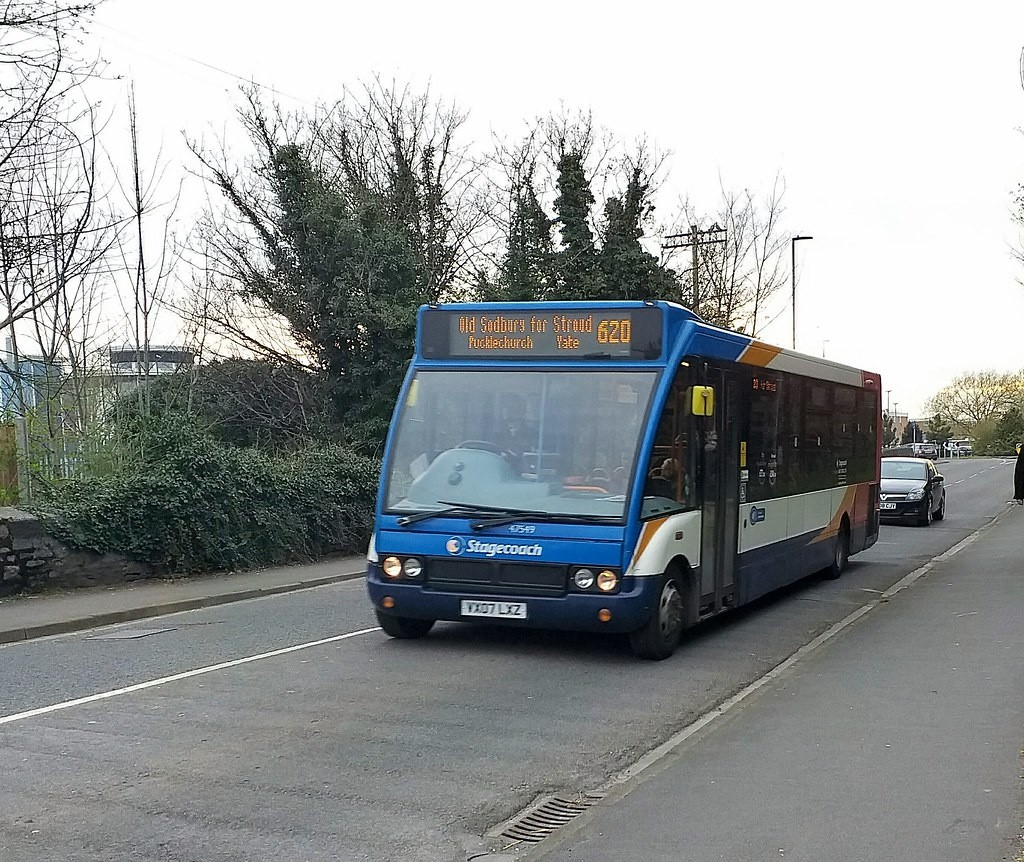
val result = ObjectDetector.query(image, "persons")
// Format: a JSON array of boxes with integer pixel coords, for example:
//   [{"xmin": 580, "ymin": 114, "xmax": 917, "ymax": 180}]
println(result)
[
  {"xmin": 595, "ymin": 447, "xmax": 689, "ymax": 501},
  {"xmin": 456, "ymin": 395, "xmax": 547, "ymax": 474}
]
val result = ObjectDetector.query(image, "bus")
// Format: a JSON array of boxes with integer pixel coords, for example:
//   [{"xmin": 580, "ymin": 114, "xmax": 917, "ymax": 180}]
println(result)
[{"xmin": 361, "ymin": 297, "xmax": 884, "ymax": 664}]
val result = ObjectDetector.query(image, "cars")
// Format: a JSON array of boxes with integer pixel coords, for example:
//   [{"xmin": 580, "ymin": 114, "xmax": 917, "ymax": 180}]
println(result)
[
  {"xmin": 915, "ymin": 447, "xmax": 937, "ymax": 461},
  {"xmin": 879, "ymin": 457, "xmax": 947, "ymax": 527}
]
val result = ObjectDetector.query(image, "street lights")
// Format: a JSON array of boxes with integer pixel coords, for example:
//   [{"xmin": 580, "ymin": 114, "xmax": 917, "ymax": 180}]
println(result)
[
  {"xmin": 885, "ymin": 390, "xmax": 891, "ymax": 415},
  {"xmin": 790, "ymin": 235, "xmax": 813, "ymax": 349},
  {"xmin": 893, "ymin": 403, "xmax": 898, "ymax": 423},
  {"xmin": 822, "ymin": 339, "xmax": 829, "ymax": 358}
]
